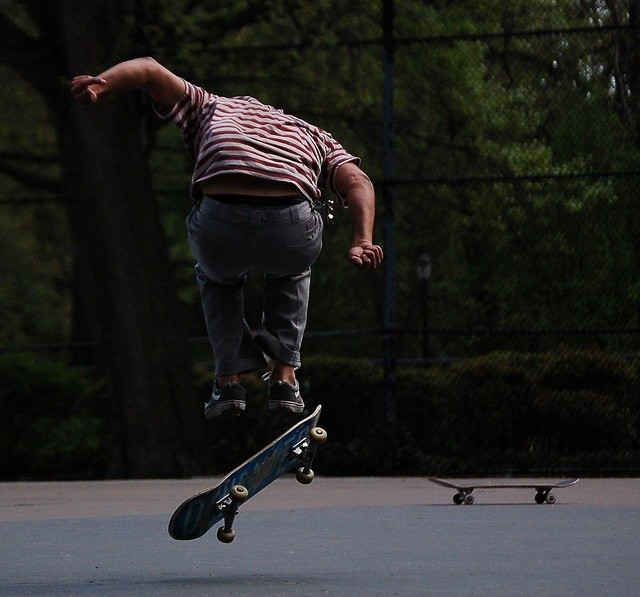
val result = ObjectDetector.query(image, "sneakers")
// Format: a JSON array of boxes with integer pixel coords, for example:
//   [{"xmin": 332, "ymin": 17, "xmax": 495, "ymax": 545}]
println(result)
[
  {"xmin": 260, "ymin": 371, "xmax": 305, "ymax": 412},
  {"xmin": 204, "ymin": 379, "xmax": 247, "ymax": 419}
]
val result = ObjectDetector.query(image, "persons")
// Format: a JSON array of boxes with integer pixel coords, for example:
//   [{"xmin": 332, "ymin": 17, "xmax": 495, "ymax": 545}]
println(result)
[{"xmin": 71, "ymin": 55, "xmax": 383, "ymax": 419}]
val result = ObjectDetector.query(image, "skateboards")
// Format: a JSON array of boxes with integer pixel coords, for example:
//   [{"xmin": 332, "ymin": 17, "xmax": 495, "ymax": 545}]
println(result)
[
  {"xmin": 428, "ymin": 476, "xmax": 579, "ymax": 504},
  {"xmin": 168, "ymin": 405, "xmax": 327, "ymax": 543}
]
